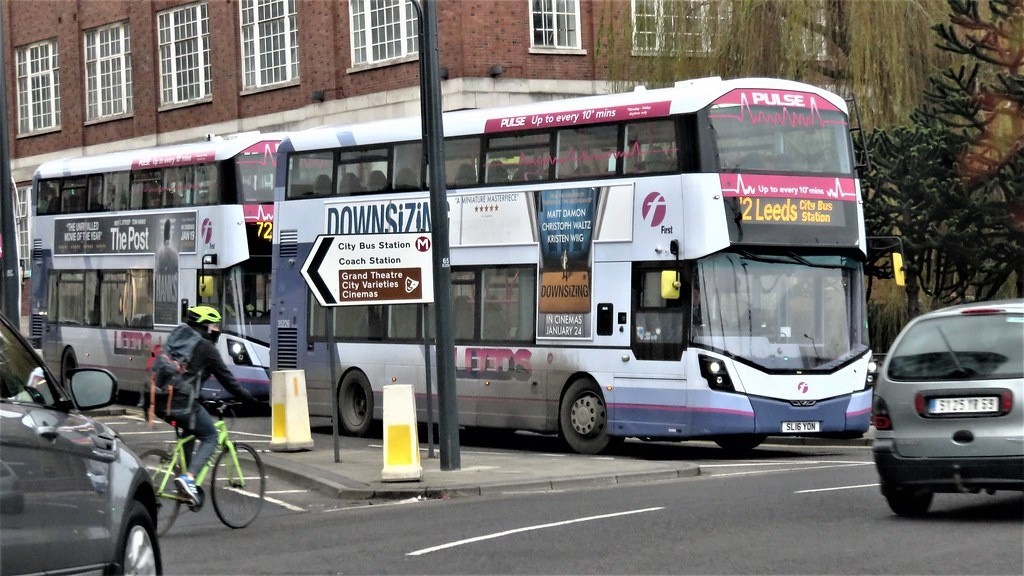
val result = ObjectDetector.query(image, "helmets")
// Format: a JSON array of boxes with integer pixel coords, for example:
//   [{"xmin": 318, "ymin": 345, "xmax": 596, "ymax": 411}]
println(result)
[{"xmin": 188, "ymin": 305, "xmax": 222, "ymax": 323}]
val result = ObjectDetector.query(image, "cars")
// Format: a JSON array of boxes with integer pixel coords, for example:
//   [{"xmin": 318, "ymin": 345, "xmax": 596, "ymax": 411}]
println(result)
[
  {"xmin": 870, "ymin": 299, "xmax": 1024, "ymax": 518},
  {"xmin": 0, "ymin": 311, "xmax": 162, "ymax": 576}
]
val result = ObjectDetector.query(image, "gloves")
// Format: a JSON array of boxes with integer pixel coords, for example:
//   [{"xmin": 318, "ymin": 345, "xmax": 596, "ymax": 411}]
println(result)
[{"xmin": 235, "ymin": 388, "xmax": 252, "ymax": 406}]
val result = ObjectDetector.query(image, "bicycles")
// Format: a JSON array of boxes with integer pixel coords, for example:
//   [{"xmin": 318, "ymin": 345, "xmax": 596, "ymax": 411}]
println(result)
[{"xmin": 138, "ymin": 399, "xmax": 265, "ymax": 539}]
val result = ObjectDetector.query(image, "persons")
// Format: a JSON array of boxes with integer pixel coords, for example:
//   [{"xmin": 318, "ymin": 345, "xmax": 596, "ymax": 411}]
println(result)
[
  {"xmin": 153, "ymin": 305, "xmax": 253, "ymax": 504},
  {"xmin": 157, "ymin": 218, "xmax": 178, "ymax": 275},
  {"xmin": 693, "ymin": 286, "xmax": 702, "ymax": 327},
  {"xmin": 561, "ymin": 249, "xmax": 569, "ymax": 281}
]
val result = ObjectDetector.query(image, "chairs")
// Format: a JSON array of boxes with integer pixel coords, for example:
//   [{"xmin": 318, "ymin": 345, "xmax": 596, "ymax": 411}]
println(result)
[
  {"xmin": 396, "ymin": 169, "xmax": 420, "ymax": 188},
  {"xmin": 314, "ymin": 174, "xmax": 332, "ymax": 194},
  {"xmin": 454, "ymin": 163, "xmax": 476, "ymax": 185},
  {"xmin": 487, "ymin": 160, "xmax": 509, "ymax": 183},
  {"xmin": 646, "ymin": 147, "xmax": 672, "ymax": 172},
  {"xmin": 340, "ymin": 173, "xmax": 361, "ymax": 194},
  {"xmin": 367, "ymin": 171, "xmax": 386, "ymax": 191}
]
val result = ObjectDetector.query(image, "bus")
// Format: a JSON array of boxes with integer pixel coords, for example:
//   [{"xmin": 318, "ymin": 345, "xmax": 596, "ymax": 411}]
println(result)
[
  {"xmin": 27, "ymin": 130, "xmax": 290, "ymax": 402},
  {"xmin": 267, "ymin": 74, "xmax": 873, "ymax": 458}
]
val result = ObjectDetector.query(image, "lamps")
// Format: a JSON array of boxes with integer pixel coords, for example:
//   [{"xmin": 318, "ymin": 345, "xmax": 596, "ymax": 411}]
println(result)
[
  {"xmin": 440, "ymin": 68, "xmax": 449, "ymax": 80},
  {"xmin": 309, "ymin": 90, "xmax": 325, "ymax": 102},
  {"xmin": 489, "ymin": 65, "xmax": 503, "ymax": 79}
]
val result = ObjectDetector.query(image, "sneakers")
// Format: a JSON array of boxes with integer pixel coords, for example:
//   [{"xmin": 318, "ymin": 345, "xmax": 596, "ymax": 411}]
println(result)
[{"xmin": 174, "ymin": 474, "xmax": 201, "ymax": 505}]
